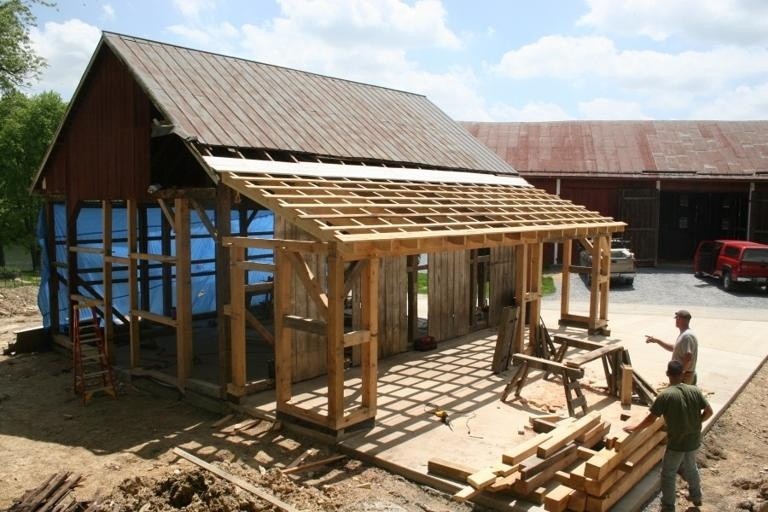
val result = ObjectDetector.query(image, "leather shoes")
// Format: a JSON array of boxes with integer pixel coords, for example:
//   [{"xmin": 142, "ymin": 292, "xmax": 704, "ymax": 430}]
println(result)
[{"xmin": 684, "ymin": 492, "xmax": 701, "ymax": 506}]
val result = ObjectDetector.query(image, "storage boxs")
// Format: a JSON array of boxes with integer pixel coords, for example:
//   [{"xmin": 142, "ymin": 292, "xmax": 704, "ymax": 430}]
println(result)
[{"xmin": 164, "ymin": 341, "xmax": 201, "ymax": 378}]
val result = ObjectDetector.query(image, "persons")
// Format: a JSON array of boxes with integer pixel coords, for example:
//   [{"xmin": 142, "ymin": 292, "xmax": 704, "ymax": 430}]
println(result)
[
  {"xmin": 623, "ymin": 360, "xmax": 713, "ymax": 505},
  {"xmin": 645, "ymin": 310, "xmax": 698, "ymax": 385}
]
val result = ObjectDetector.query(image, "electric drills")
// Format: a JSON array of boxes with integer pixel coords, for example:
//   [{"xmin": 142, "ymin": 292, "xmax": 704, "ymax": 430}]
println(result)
[{"xmin": 435, "ymin": 410, "xmax": 455, "ymax": 432}]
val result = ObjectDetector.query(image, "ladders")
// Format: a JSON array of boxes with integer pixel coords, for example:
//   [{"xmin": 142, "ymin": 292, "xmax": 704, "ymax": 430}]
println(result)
[{"xmin": 72, "ymin": 301, "xmax": 119, "ymax": 406}]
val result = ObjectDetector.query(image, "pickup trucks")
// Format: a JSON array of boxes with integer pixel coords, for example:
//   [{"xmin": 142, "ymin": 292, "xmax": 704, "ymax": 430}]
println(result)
[{"xmin": 577, "ymin": 239, "xmax": 638, "ymax": 289}]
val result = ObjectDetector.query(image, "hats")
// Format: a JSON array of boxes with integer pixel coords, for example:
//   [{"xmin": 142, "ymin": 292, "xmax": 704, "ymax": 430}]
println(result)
[{"xmin": 675, "ymin": 310, "xmax": 691, "ymax": 319}]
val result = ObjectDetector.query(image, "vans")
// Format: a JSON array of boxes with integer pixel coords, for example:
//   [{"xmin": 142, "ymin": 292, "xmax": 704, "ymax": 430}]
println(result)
[{"xmin": 692, "ymin": 238, "xmax": 768, "ymax": 295}]
